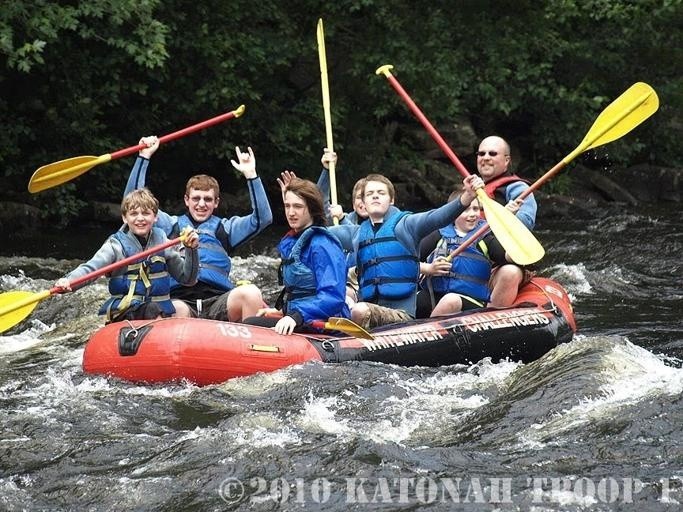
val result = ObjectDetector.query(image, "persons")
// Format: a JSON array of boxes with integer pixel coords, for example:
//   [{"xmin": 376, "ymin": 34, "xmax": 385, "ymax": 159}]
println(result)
[{"xmin": 53, "ymin": 136, "xmax": 538, "ymax": 336}]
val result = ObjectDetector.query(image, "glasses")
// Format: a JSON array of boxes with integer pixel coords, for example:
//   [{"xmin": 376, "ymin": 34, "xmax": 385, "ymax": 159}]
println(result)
[
  {"xmin": 187, "ymin": 195, "xmax": 215, "ymax": 203},
  {"xmin": 475, "ymin": 150, "xmax": 507, "ymax": 157}
]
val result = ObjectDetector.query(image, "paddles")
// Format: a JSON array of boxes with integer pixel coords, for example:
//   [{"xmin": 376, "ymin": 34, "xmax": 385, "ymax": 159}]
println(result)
[
  {"xmin": 0, "ymin": 235, "xmax": 188, "ymax": 333},
  {"xmin": 262, "ymin": 313, "xmax": 374, "ymax": 340},
  {"xmin": 375, "ymin": 64, "xmax": 545, "ymax": 266},
  {"xmin": 314, "ymin": 18, "xmax": 338, "ymax": 225},
  {"xmin": 28, "ymin": 104, "xmax": 245, "ymax": 193},
  {"xmin": 446, "ymin": 81, "xmax": 660, "ymax": 264}
]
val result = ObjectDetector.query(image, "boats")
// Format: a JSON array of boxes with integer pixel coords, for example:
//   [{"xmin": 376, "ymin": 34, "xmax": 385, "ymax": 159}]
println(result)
[{"xmin": 82, "ymin": 277, "xmax": 577, "ymax": 385}]
